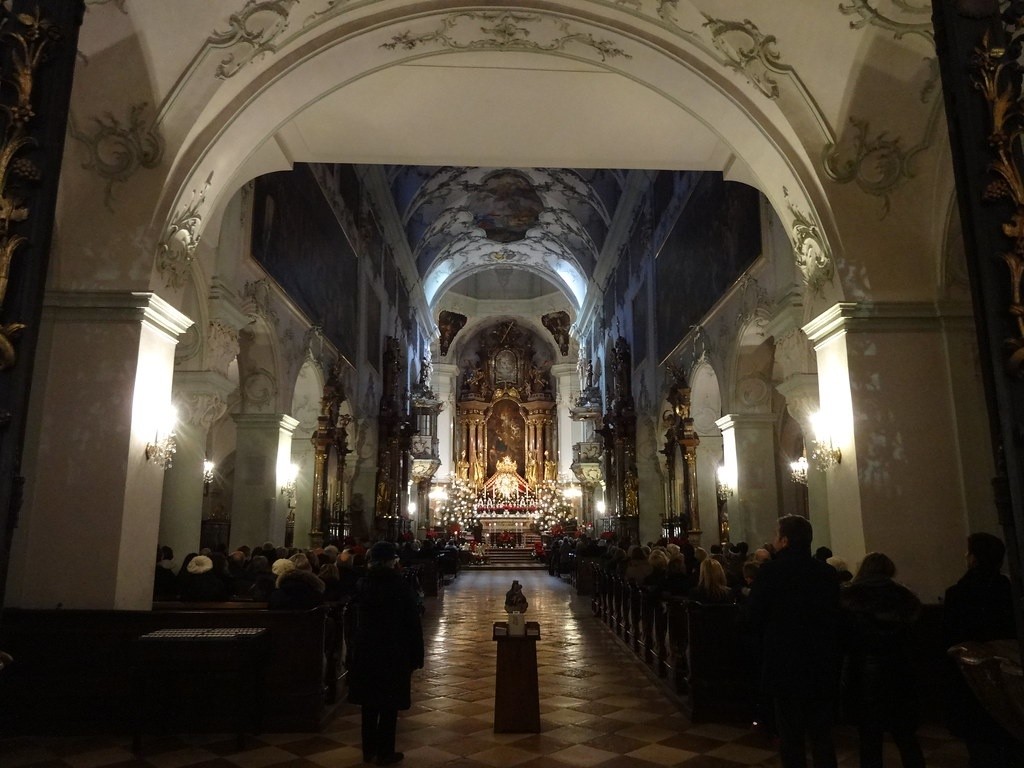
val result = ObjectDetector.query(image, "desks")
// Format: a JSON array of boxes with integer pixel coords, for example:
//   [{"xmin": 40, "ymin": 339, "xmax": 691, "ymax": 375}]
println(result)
[{"xmin": 490, "ymin": 532, "xmax": 522, "ymax": 548}]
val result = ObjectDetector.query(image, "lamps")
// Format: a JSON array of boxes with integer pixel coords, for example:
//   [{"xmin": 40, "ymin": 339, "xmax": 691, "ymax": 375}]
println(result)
[
  {"xmin": 281, "ymin": 480, "xmax": 295, "ymax": 494},
  {"xmin": 146, "ymin": 435, "xmax": 177, "ymax": 471},
  {"xmin": 812, "ymin": 437, "xmax": 841, "ymax": 471},
  {"xmin": 718, "ymin": 480, "xmax": 733, "ymax": 502}
]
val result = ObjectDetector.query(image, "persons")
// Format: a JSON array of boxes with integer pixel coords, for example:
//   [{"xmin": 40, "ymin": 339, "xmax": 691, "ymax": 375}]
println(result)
[
  {"xmin": 342, "ymin": 541, "xmax": 424, "ymax": 764},
  {"xmin": 747, "ymin": 512, "xmax": 848, "ymax": 768},
  {"xmin": 941, "ymin": 531, "xmax": 1024, "ymax": 768},
  {"xmin": 837, "ymin": 551, "xmax": 928, "ymax": 768},
  {"xmin": 155, "ymin": 525, "xmax": 777, "ymax": 704}
]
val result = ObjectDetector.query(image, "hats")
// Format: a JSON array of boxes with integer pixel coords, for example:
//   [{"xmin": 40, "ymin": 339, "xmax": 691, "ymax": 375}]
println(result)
[
  {"xmin": 272, "ymin": 558, "xmax": 295, "ymax": 575},
  {"xmin": 187, "ymin": 555, "xmax": 213, "ymax": 574}
]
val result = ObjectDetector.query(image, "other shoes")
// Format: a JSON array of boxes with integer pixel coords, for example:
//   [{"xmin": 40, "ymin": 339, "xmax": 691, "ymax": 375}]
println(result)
[{"xmin": 364, "ymin": 752, "xmax": 404, "ymax": 765}]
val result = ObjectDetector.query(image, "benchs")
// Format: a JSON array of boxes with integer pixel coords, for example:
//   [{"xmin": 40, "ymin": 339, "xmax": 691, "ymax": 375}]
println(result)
[
  {"xmin": 551, "ymin": 553, "xmax": 954, "ymax": 723},
  {"xmin": 0, "ymin": 552, "xmax": 461, "ymax": 733}
]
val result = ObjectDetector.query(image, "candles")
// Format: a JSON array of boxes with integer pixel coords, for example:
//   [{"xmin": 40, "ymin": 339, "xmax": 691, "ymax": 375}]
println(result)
[
  {"xmin": 484, "ymin": 485, "xmax": 486, "ymax": 499},
  {"xmin": 517, "ymin": 485, "xmax": 519, "ymax": 499},
  {"xmin": 526, "ymin": 485, "xmax": 528, "ymax": 500},
  {"xmin": 493, "ymin": 484, "xmax": 495, "ymax": 502},
  {"xmin": 475, "ymin": 483, "xmax": 477, "ymax": 497},
  {"xmin": 535, "ymin": 485, "xmax": 537, "ymax": 498}
]
